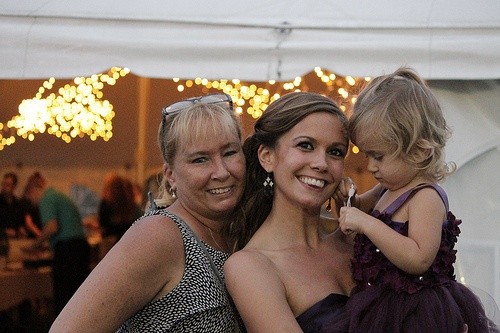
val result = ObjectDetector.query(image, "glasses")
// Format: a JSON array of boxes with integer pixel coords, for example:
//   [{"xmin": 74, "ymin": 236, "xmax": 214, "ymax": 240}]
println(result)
[{"xmin": 161, "ymin": 93, "xmax": 233, "ymax": 142}]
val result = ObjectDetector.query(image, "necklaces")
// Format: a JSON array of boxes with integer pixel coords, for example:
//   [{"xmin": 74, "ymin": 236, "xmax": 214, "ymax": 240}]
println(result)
[{"xmin": 208, "ymin": 227, "xmax": 226, "ymax": 253}]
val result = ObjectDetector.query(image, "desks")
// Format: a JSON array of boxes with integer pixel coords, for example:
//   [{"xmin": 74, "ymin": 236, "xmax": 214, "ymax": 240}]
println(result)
[{"xmin": 0, "ymin": 254, "xmax": 54, "ymax": 325}]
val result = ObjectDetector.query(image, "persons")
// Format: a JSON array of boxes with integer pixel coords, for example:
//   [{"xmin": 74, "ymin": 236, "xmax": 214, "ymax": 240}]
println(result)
[
  {"xmin": 224, "ymin": 93, "xmax": 358, "ymax": 333},
  {"xmin": 47, "ymin": 94, "xmax": 251, "ymax": 333},
  {"xmin": 329, "ymin": 66, "xmax": 490, "ymax": 333},
  {"xmin": 0, "ymin": 172, "xmax": 173, "ymax": 333}
]
